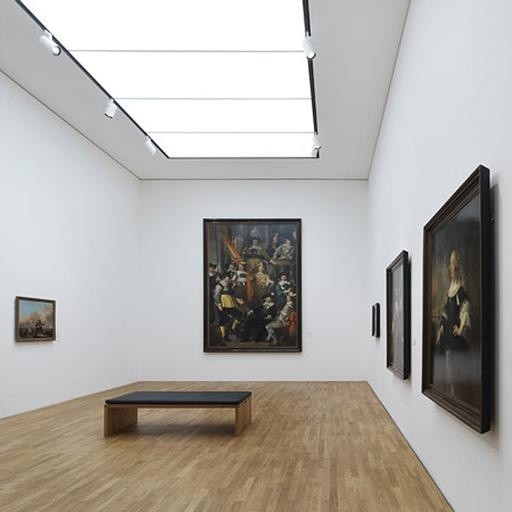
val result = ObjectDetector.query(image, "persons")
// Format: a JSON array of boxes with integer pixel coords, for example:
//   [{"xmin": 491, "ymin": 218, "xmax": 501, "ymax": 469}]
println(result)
[
  {"xmin": 429, "ymin": 246, "xmax": 471, "ymax": 401},
  {"xmin": 209, "ymin": 222, "xmax": 298, "ymax": 347}
]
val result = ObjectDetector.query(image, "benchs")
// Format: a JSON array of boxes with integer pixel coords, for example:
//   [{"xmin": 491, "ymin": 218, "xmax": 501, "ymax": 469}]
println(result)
[{"xmin": 103, "ymin": 391, "xmax": 252, "ymax": 436}]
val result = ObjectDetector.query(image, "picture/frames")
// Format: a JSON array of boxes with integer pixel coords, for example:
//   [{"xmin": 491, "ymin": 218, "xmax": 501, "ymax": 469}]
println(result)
[
  {"xmin": 201, "ymin": 218, "xmax": 303, "ymax": 354},
  {"xmin": 384, "ymin": 163, "xmax": 494, "ymax": 435},
  {"xmin": 14, "ymin": 295, "xmax": 57, "ymax": 343}
]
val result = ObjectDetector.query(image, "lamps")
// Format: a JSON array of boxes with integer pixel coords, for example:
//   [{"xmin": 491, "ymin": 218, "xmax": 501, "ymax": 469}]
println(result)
[
  {"xmin": 302, "ymin": 33, "xmax": 317, "ymax": 60},
  {"xmin": 311, "ymin": 130, "xmax": 323, "ymax": 152},
  {"xmin": 144, "ymin": 137, "xmax": 158, "ymax": 158},
  {"xmin": 105, "ymin": 97, "xmax": 118, "ymax": 120},
  {"xmin": 39, "ymin": 30, "xmax": 61, "ymax": 58}
]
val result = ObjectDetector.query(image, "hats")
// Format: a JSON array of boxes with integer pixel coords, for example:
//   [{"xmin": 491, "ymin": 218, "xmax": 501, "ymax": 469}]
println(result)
[
  {"xmin": 264, "ymin": 291, "xmax": 273, "ymax": 299},
  {"xmin": 209, "ymin": 260, "xmax": 246, "ymax": 279},
  {"xmin": 257, "ymin": 262, "xmax": 267, "ymax": 269},
  {"xmin": 279, "ymin": 272, "xmax": 289, "ymax": 278},
  {"xmin": 248, "ymin": 232, "xmax": 280, "ymax": 242}
]
